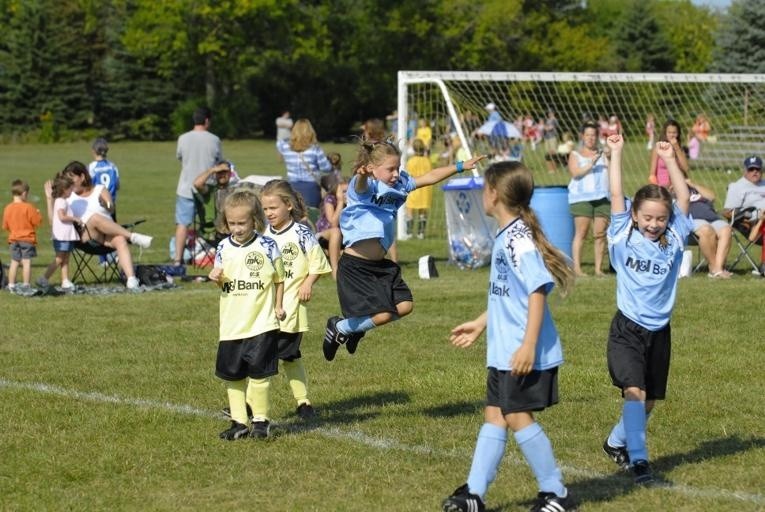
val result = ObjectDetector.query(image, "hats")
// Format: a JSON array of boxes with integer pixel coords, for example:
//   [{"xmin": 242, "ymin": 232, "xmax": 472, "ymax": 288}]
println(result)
[{"xmin": 744, "ymin": 156, "xmax": 762, "ymax": 169}]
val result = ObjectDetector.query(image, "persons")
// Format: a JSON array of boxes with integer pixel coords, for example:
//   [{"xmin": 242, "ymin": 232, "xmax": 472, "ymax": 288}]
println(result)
[
  {"xmin": 601, "ymin": 134, "xmax": 696, "ymax": 483},
  {"xmin": 691, "ymin": 114, "xmax": 711, "ymax": 142},
  {"xmin": 1, "ymin": 178, "xmax": 41, "ymax": 293},
  {"xmin": 193, "ymin": 159, "xmax": 234, "ymax": 248},
  {"xmin": 172, "ymin": 108, "xmax": 223, "ymax": 266},
  {"xmin": 323, "ymin": 141, "xmax": 489, "ymax": 362},
  {"xmin": 322, "ymin": 151, "xmax": 345, "ymax": 193},
  {"xmin": 648, "ymin": 119, "xmax": 689, "ymax": 189},
  {"xmin": 207, "ymin": 190, "xmax": 287, "ymax": 440},
  {"xmin": 35, "ymin": 175, "xmax": 82, "ymax": 290},
  {"xmin": 364, "ymin": 102, "xmax": 544, "ymax": 157},
  {"xmin": 544, "ymin": 109, "xmax": 558, "ymax": 151},
  {"xmin": 597, "ymin": 115, "xmax": 608, "ymax": 147},
  {"xmin": 404, "ymin": 138, "xmax": 433, "ymax": 240},
  {"xmin": 316, "ymin": 175, "xmax": 351, "ymax": 283},
  {"xmin": 220, "ymin": 179, "xmax": 333, "ymax": 419},
  {"xmin": 721, "ymin": 155, "xmax": 765, "ymax": 227},
  {"xmin": 645, "ymin": 112, "xmax": 656, "ymax": 149},
  {"xmin": 682, "ymin": 129, "xmax": 699, "ymax": 160},
  {"xmin": 275, "ymin": 118, "xmax": 333, "ymax": 208},
  {"xmin": 86, "ymin": 138, "xmax": 121, "ymax": 267},
  {"xmin": 567, "ymin": 123, "xmax": 610, "ymax": 278},
  {"xmin": 544, "ymin": 130, "xmax": 576, "ymax": 173},
  {"xmin": 608, "ymin": 115, "xmax": 623, "ymax": 136},
  {"xmin": 748, "ymin": 211, "xmax": 765, "ymax": 276},
  {"xmin": 275, "ymin": 110, "xmax": 294, "ymax": 163},
  {"xmin": 441, "ymin": 160, "xmax": 574, "ymax": 512},
  {"xmin": 667, "ymin": 168, "xmax": 735, "ymax": 279},
  {"xmin": 44, "ymin": 161, "xmax": 154, "ymax": 290}
]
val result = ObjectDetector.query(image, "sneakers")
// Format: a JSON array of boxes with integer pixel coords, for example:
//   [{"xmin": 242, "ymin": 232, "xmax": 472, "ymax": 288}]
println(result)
[
  {"xmin": 630, "ymin": 459, "xmax": 654, "ymax": 486},
  {"xmin": 61, "ymin": 279, "xmax": 74, "ymax": 288},
  {"xmin": 297, "ymin": 403, "xmax": 313, "ymax": 417},
  {"xmin": 127, "ymin": 276, "xmax": 139, "ymax": 289},
  {"xmin": 220, "ymin": 420, "xmax": 250, "ymax": 441},
  {"xmin": 35, "ymin": 275, "xmax": 49, "ymax": 287},
  {"xmin": 527, "ymin": 491, "xmax": 577, "ymax": 512},
  {"xmin": 221, "ymin": 403, "xmax": 252, "ymax": 417},
  {"xmin": 323, "ymin": 316, "xmax": 343, "ymax": 361},
  {"xmin": 249, "ymin": 417, "xmax": 270, "ymax": 439},
  {"xmin": 130, "ymin": 232, "xmax": 154, "ymax": 248},
  {"xmin": 346, "ymin": 331, "xmax": 365, "ymax": 354},
  {"xmin": 442, "ymin": 483, "xmax": 485, "ymax": 512},
  {"xmin": 602, "ymin": 437, "xmax": 629, "ymax": 469}
]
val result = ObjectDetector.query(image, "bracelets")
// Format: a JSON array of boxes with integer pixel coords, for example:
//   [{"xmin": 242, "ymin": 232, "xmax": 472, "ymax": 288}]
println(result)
[{"xmin": 456, "ymin": 160, "xmax": 464, "ymax": 173}]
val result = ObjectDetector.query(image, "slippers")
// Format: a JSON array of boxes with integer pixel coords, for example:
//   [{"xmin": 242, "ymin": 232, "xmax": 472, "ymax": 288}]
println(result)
[{"xmin": 707, "ymin": 270, "xmax": 733, "ymax": 278}]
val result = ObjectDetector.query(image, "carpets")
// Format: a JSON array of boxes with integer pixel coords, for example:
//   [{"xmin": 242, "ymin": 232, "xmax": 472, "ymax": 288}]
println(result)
[{"xmin": 10, "ymin": 281, "xmax": 175, "ymax": 297}]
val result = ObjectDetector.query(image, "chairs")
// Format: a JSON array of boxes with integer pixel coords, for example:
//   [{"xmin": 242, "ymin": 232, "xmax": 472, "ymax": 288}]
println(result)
[
  {"xmin": 71, "ymin": 240, "xmax": 120, "ymax": 286},
  {"xmin": 192, "ymin": 191, "xmax": 217, "ymax": 269},
  {"xmin": 729, "ymin": 206, "xmax": 763, "ymax": 276},
  {"xmin": 683, "ymin": 231, "xmax": 711, "ymax": 274}
]
